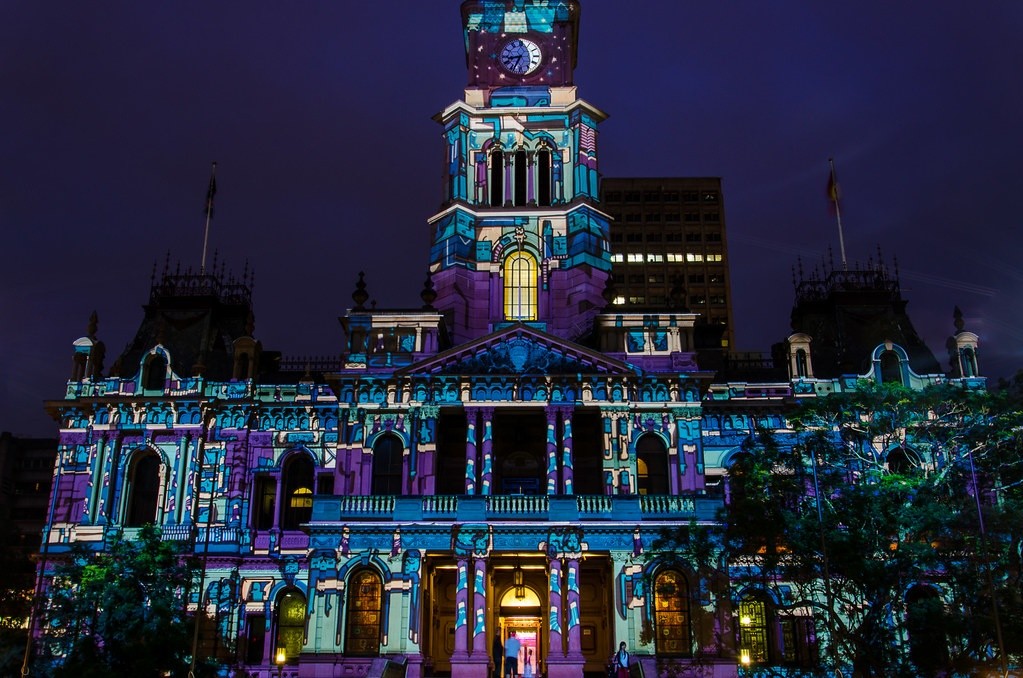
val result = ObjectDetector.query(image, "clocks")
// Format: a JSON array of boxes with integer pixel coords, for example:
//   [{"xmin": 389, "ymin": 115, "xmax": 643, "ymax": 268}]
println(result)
[{"xmin": 500, "ymin": 38, "xmax": 542, "ymax": 75}]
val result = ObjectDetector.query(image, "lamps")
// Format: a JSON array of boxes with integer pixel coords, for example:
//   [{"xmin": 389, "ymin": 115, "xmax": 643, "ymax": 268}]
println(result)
[{"xmin": 513, "ymin": 557, "xmax": 525, "ymax": 601}]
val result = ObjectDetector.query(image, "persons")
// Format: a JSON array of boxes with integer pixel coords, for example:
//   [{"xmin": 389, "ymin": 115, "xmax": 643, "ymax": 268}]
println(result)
[
  {"xmin": 616, "ymin": 641, "xmax": 631, "ymax": 678},
  {"xmin": 492, "ymin": 627, "xmax": 520, "ymax": 678}
]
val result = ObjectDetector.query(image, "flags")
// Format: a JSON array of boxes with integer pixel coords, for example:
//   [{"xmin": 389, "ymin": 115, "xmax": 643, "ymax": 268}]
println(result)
[
  {"xmin": 826, "ymin": 164, "xmax": 841, "ymax": 218},
  {"xmin": 205, "ymin": 164, "xmax": 218, "ymax": 221}
]
